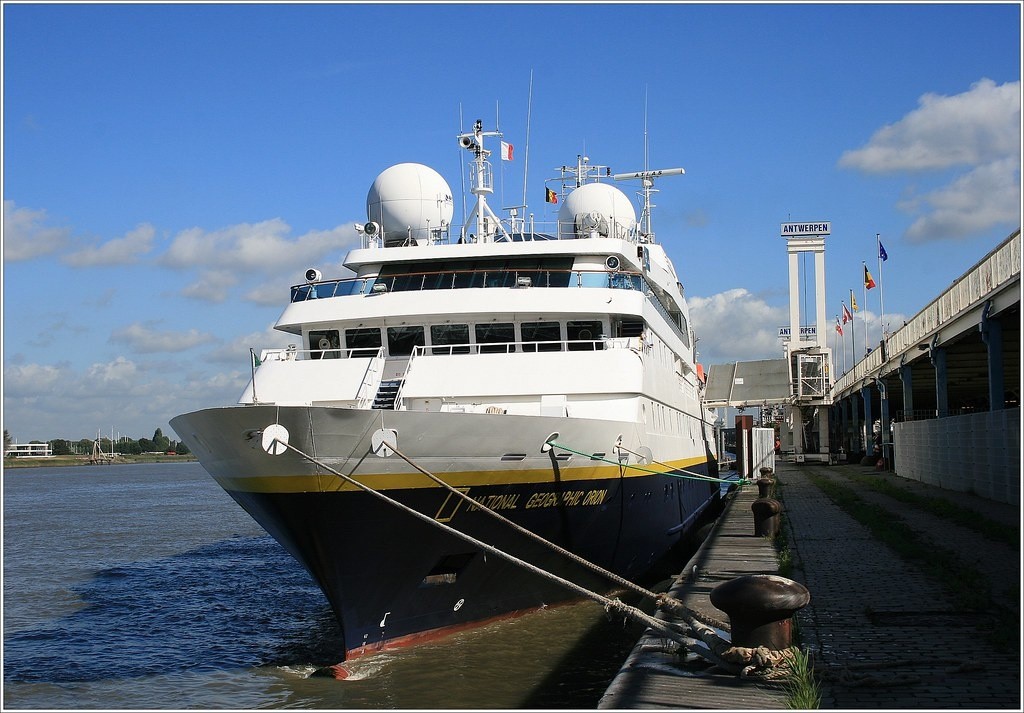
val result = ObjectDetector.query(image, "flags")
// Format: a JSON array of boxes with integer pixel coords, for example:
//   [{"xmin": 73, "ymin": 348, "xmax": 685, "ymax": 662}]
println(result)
[
  {"xmin": 852, "ymin": 295, "xmax": 858, "ymax": 310},
  {"xmin": 879, "ymin": 242, "xmax": 887, "ymax": 261},
  {"xmin": 865, "ymin": 267, "xmax": 876, "ymax": 290},
  {"xmin": 500, "ymin": 141, "xmax": 514, "ymax": 160},
  {"xmin": 545, "ymin": 188, "xmax": 557, "ymax": 204},
  {"xmin": 836, "ymin": 320, "xmax": 843, "ymax": 336},
  {"xmin": 843, "ymin": 306, "xmax": 852, "ymax": 324}
]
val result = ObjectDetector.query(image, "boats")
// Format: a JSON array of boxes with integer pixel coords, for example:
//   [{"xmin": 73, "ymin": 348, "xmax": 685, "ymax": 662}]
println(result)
[{"xmin": 169, "ymin": 66, "xmax": 720, "ymax": 664}]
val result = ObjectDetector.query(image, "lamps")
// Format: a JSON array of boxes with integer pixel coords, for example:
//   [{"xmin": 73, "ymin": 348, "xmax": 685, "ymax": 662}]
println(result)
[{"xmin": 917, "ymin": 344, "xmax": 930, "ymax": 351}]
